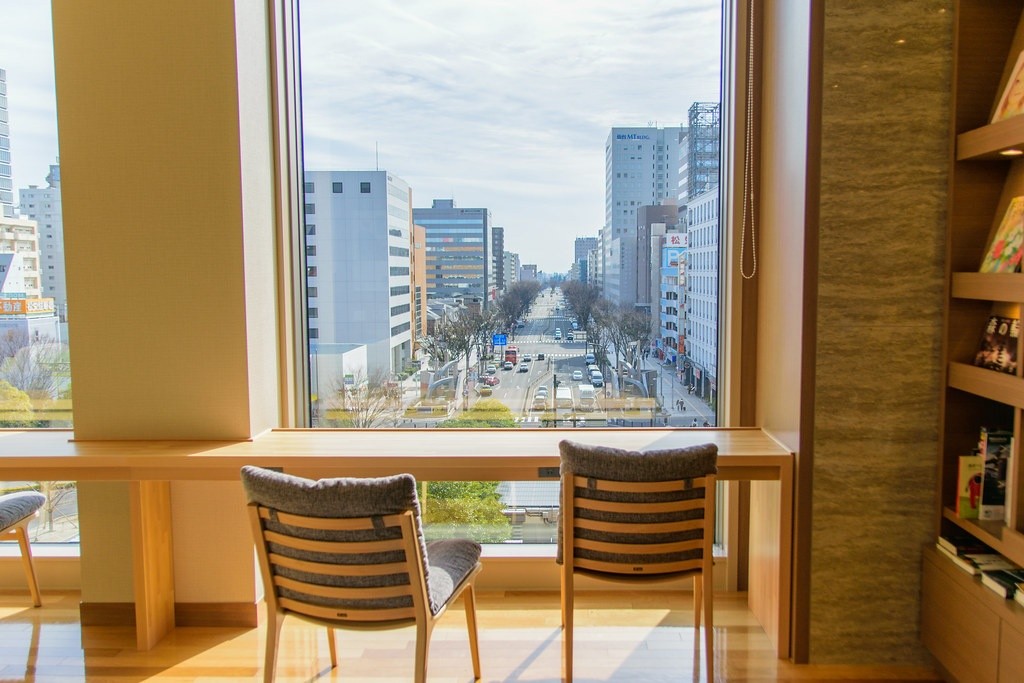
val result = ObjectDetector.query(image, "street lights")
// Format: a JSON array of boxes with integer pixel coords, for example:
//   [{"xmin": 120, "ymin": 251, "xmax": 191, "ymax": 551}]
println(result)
[
  {"xmin": 399, "ymin": 373, "xmax": 406, "ymax": 406},
  {"xmin": 657, "ymin": 362, "xmax": 666, "ymax": 396},
  {"xmin": 668, "ymin": 372, "xmax": 678, "ymax": 409}
]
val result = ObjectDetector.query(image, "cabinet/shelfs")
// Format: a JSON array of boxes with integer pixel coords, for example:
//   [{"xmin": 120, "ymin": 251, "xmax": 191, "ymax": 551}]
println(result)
[{"xmin": 919, "ymin": 0, "xmax": 1024, "ymax": 683}]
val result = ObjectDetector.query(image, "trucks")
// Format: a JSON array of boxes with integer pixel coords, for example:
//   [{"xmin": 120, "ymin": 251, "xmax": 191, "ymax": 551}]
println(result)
[
  {"xmin": 579, "ymin": 385, "xmax": 595, "ymax": 412},
  {"xmin": 551, "ymin": 387, "xmax": 574, "ymax": 414}
]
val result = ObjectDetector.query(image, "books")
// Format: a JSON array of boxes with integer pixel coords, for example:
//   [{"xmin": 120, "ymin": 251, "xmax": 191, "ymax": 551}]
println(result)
[
  {"xmin": 936, "ymin": 542, "xmax": 1024, "ymax": 575},
  {"xmin": 972, "ymin": 315, "xmax": 1020, "ymax": 376},
  {"xmin": 991, "ymin": 50, "xmax": 1024, "ymax": 155},
  {"xmin": 956, "ymin": 427, "xmax": 1014, "ymax": 528},
  {"xmin": 979, "ymin": 197, "xmax": 1024, "ymax": 273},
  {"xmin": 981, "ymin": 569, "xmax": 1024, "ymax": 608},
  {"xmin": 938, "ymin": 535, "xmax": 1003, "ymax": 556}
]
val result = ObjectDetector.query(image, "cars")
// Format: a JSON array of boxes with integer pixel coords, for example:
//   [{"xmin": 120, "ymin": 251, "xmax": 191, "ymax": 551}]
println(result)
[
  {"xmin": 538, "ymin": 353, "xmax": 544, "ymax": 360},
  {"xmin": 504, "ymin": 361, "xmax": 513, "ymax": 369},
  {"xmin": 480, "ymin": 357, "xmax": 503, "ymax": 385},
  {"xmin": 524, "ymin": 354, "xmax": 531, "ymax": 361},
  {"xmin": 533, "ymin": 386, "xmax": 548, "ymax": 414},
  {"xmin": 567, "ymin": 317, "xmax": 582, "ymax": 340},
  {"xmin": 574, "ymin": 371, "xmax": 583, "ymax": 380},
  {"xmin": 520, "ymin": 364, "xmax": 528, "ymax": 372}
]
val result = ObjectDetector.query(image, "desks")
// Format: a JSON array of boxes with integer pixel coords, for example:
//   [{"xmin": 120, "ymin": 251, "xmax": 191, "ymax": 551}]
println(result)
[{"xmin": 0, "ymin": 427, "xmax": 797, "ymax": 660}]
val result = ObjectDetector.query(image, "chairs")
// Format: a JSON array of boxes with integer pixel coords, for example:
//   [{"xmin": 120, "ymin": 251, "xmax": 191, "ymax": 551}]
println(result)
[
  {"xmin": 555, "ymin": 439, "xmax": 720, "ymax": 683},
  {"xmin": 0, "ymin": 491, "xmax": 47, "ymax": 607},
  {"xmin": 240, "ymin": 465, "xmax": 483, "ymax": 683}
]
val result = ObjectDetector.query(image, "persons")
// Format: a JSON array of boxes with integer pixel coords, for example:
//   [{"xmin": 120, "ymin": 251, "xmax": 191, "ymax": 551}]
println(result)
[
  {"xmin": 703, "ymin": 420, "xmax": 709, "ymax": 427},
  {"xmin": 676, "ymin": 400, "xmax": 679, "ymax": 411},
  {"xmin": 692, "ymin": 418, "xmax": 698, "ymax": 427},
  {"xmin": 679, "ymin": 399, "xmax": 684, "ymax": 410}
]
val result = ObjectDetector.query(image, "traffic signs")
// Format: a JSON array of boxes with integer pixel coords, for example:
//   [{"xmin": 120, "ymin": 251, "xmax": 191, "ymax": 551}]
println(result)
[{"xmin": 493, "ymin": 333, "xmax": 506, "ymax": 345}]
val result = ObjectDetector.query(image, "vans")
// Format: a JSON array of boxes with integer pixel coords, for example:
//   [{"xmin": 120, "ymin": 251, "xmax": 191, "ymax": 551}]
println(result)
[
  {"xmin": 556, "ymin": 327, "xmax": 561, "ymax": 339},
  {"xmin": 585, "ymin": 354, "xmax": 603, "ymax": 387}
]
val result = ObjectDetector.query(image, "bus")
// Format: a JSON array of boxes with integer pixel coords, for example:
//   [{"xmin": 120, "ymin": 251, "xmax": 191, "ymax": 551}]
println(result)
[{"xmin": 505, "ymin": 346, "xmax": 519, "ymax": 364}]
